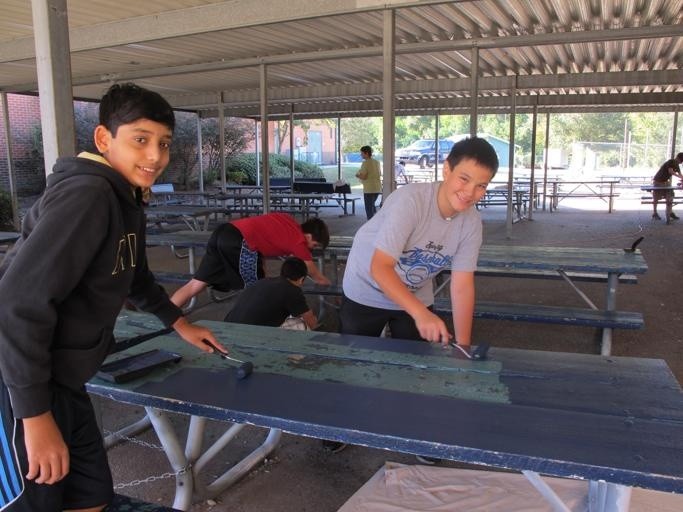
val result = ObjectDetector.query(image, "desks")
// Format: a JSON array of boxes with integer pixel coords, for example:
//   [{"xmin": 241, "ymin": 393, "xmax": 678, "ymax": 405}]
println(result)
[
  {"xmin": 102, "ymin": 307, "xmax": 187, "ymax": 450},
  {"xmin": 315, "ymin": 235, "xmax": 646, "ymax": 307},
  {"xmin": 86, "ymin": 321, "xmax": 681, "ymax": 511}
]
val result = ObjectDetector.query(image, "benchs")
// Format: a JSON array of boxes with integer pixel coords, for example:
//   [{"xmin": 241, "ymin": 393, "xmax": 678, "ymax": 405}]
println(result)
[
  {"xmin": 474, "ymin": 175, "xmax": 681, "ymax": 222},
  {"xmin": 297, "ymin": 283, "xmax": 645, "ymax": 356},
  {"xmin": 141, "ymin": 175, "xmax": 443, "ymax": 252},
  {"xmin": 152, "ymin": 270, "xmax": 196, "ymax": 286}
]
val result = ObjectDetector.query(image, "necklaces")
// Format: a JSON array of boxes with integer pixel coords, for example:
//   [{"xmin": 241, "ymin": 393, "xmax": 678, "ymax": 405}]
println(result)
[{"xmin": 443, "ymin": 211, "xmax": 460, "ymax": 221}]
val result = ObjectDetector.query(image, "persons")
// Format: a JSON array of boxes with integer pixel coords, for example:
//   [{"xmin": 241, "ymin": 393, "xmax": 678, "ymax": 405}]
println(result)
[
  {"xmin": 651, "ymin": 152, "xmax": 683, "ymax": 220},
  {"xmin": 0, "ymin": 82, "xmax": 228, "ymax": 511},
  {"xmin": 170, "ymin": 213, "xmax": 331, "ymax": 331},
  {"xmin": 321, "ymin": 139, "xmax": 499, "ymax": 466},
  {"xmin": 355, "ymin": 145, "xmax": 411, "ymax": 220}
]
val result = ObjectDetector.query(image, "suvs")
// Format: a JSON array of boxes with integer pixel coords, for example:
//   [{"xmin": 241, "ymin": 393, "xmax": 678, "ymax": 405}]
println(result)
[{"xmin": 395, "ymin": 136, "xmax": 458, "ymax": 168}]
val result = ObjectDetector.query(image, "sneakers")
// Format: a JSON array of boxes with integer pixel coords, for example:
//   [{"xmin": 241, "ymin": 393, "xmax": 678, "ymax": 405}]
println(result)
[
  {"xmin": 652, "ymin": 213, "xmax": 680, "ymax": 222},
  {"xmin": 320, "ymin": 440, "xmax": 346, "ymax": 454},
  {"xmin": 416, "ymin": 455, "xmax": 441, "ymax": 465}
]
